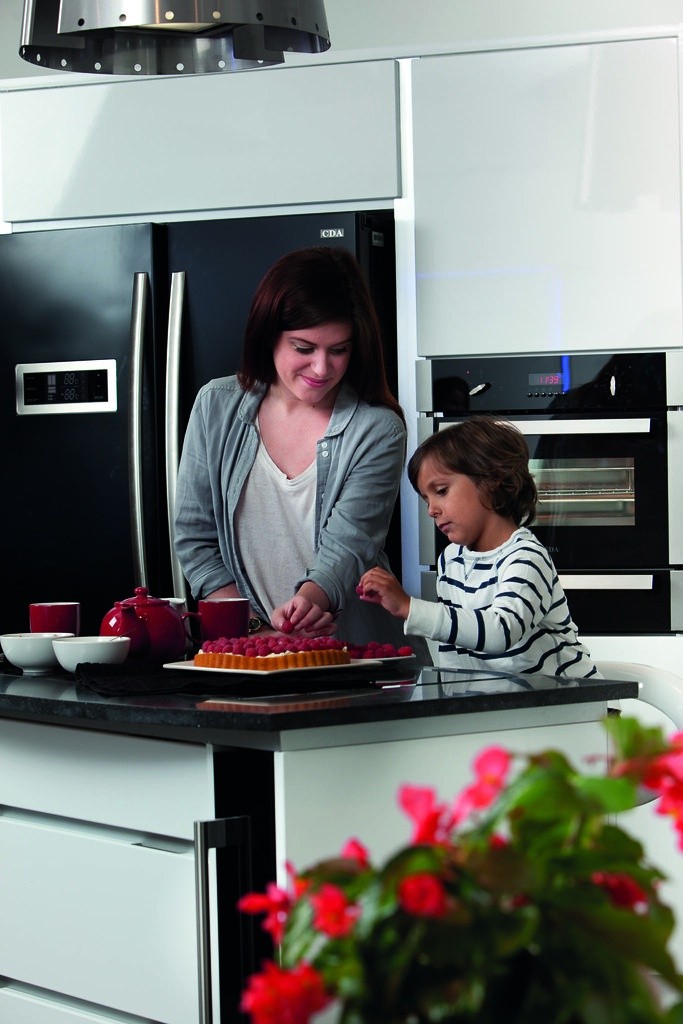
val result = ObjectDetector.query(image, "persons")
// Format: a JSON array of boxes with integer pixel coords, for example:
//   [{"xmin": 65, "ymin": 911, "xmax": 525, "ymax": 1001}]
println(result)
[
  {"xmin": 356, "ymin": 417, "xmax": 622, "ymax": 720},
  {"xmin": 172, "ymin": 246, "xmax": 432, "ymax": 676}
]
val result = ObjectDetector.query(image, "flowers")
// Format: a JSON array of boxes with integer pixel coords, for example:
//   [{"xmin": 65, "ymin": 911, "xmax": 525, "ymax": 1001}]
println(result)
[{"xmin": 238, "ymin": 709, "xmax": 683, "ymax": 1024}]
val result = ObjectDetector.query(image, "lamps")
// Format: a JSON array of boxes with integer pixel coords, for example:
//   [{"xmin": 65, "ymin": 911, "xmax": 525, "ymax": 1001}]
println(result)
[{"xmin": 18, "ymin": 1, "xmax": 330, "ymax": 77}]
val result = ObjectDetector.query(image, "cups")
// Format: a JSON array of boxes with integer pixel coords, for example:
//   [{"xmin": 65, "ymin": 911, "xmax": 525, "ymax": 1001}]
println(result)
[
  {"xmin": 157, "ymin": 597, "xmax": 187, "ymax": 619},
  {"xmin": 29, "ymin": 602, "xmax": 81, "ymax": 637},
  {"xmin": 182, "ymin": 597, "xmax": 250, "ymax": 645}
]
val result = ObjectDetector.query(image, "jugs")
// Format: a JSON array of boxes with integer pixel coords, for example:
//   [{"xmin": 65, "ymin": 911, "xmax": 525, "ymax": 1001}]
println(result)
[{"xmin": 100, "ymin": 588, "xmax": 186, "ymax": 665}]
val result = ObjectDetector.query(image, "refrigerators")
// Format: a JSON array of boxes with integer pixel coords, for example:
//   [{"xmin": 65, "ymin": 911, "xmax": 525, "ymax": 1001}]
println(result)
[{"xmin": 0, "ymin": 209, "xmax": 412, "ymax": 650}]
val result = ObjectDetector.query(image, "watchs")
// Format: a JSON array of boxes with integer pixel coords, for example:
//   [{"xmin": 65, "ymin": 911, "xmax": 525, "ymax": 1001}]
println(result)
[{"xmin": 249, "ymin": 616, "xmax": 267, "ymax": 631}]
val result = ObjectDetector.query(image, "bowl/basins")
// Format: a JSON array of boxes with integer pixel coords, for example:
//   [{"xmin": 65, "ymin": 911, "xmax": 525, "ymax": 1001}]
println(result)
[
  {"xmin": 0, "ymin": 632, "xmax": 75, "ymax": 673},
  {"xmin": 52, "ymin": 636, "xmax": 131, "ymax": 673}
]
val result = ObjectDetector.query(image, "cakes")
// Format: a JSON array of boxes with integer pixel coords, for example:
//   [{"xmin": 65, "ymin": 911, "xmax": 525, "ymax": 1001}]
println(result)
[{"xmin": 194, "ymin": 636, "xmax": 351, "ymax": 670}]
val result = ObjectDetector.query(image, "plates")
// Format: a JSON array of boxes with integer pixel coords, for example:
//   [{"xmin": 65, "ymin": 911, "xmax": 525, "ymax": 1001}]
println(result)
[{"xmin": 162, "ymin": 652, "xmax": 416, "ymax": 679}]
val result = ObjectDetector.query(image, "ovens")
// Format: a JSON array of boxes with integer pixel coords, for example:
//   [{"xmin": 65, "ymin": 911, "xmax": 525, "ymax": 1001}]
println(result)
[{"xmin": 412, "ymin": 350, "xmax": 683, "ymax": 636}]
